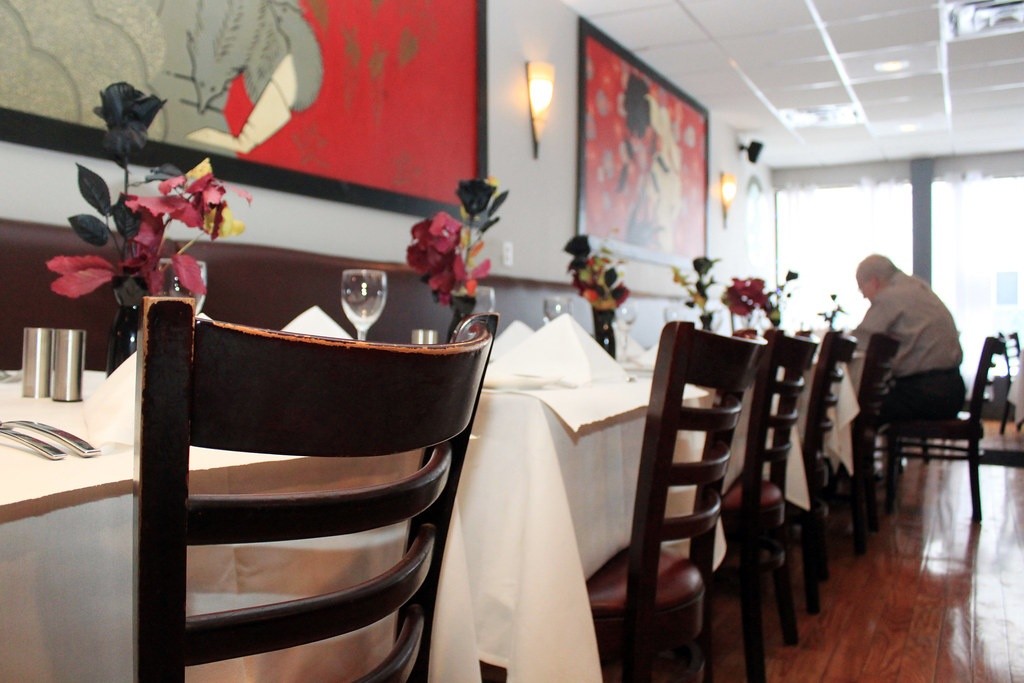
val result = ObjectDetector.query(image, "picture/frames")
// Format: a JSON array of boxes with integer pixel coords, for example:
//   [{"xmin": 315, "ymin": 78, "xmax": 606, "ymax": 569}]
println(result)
[
  {"xmin": 0, "ymin": 0, "xmax": 489, "ymax": 230},
  {"xmin": 577, "ymin": 17, "xmax": 710, "ymax": 270}
]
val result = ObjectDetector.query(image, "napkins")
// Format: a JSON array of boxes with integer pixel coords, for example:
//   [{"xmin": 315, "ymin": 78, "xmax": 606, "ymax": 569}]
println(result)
[
  {"xmin": 485, "ymin": 315, "xmax": 652, "ymax": 431},
  {"xmin": 86, "ymin": 306, "xmax": 349, "ymax": 464}
]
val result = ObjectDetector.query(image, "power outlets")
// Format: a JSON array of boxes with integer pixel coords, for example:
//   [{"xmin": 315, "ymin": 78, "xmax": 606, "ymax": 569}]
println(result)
[{"xmin": 503, "ymin": 242, "xmax": 514, "ymax": 265}]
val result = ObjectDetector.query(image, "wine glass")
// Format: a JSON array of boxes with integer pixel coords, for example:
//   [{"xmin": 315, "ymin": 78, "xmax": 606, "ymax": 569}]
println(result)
[{"xmin": 340, "ymin": 268, "xmax": 389, "ymax": 342}]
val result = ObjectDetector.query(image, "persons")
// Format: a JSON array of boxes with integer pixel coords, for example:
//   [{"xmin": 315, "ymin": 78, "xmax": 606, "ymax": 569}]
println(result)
[{"xmin": 849, "ymin": 253, "xmax": 966, "ymax": 426}]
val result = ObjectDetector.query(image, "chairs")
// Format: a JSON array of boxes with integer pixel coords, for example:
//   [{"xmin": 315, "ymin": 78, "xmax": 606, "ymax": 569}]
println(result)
[{"xmin": 118, "ymin": 289, "xmax": 1024, "ymax": 683}]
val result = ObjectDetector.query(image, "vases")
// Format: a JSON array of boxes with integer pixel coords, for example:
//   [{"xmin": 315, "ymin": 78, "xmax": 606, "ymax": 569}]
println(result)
[
  {"xmin": 593, "ymin": 307, "xmax": 618, "ymax": 359},
  {"xmin": 107, "ymin": 303, "xmax": 137, "ymax": 377},
  {"xmin": 698, "ymin": 313, "xmax": 716, "ymax": 334},
  {"xmin": 447, "ymin": 295, "xmax": 476, "ymax": 344}
]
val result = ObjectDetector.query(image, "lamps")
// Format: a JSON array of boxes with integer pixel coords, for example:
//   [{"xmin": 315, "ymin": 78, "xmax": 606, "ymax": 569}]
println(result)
[
  {"xmin": 722, "ymin": 172, "xmax": 737, "ymax": 229},
  {"xmin": 525, "ymin": 60, "xmax": 555, "ymax": 161}
]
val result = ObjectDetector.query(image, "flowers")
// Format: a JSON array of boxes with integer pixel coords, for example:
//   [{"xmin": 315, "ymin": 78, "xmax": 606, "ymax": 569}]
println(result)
[
  {"xmin": 673, "ymin": 255, "xmax": 796, "ymax": 315},
  {"xmin": 46, "ymin": 80, "xmax": 240, "ymax": 312},
  {"xmin": 408, "ymin": 179, "xmax": 509, "ymax": 315},
  {"xmin": 564, "ymin": 231, "xmax": 630, "ymax": 315}
]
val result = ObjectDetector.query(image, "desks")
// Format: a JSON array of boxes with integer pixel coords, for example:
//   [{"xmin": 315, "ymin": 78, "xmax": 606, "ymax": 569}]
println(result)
[{"xmin": 0, "ymin": 306, "xmax": 870, "ymax": 683}]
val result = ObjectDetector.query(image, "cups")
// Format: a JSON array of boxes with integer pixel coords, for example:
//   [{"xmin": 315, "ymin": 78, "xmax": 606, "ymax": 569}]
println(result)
[
  {"xmin": 21, "ymin": 326, "xmax": 89, "ymax": 402},
  {"xmin": 543, "ymin": 295, "xmax": 573, "ymax": 324},
  {"xmin": 664, "ymin": 307, "xmax": 684, "ymax": 324},
  {"xmin": 157, "ymin": 258, "xmax": 208, "ymax": 314},
  {"xmin": 614, "ymin": 305, "xmax": 637, "ymax": 326},
  {"xmin": 472, "ymin": 284, "xmax": 497, "ymax": 316}
]
